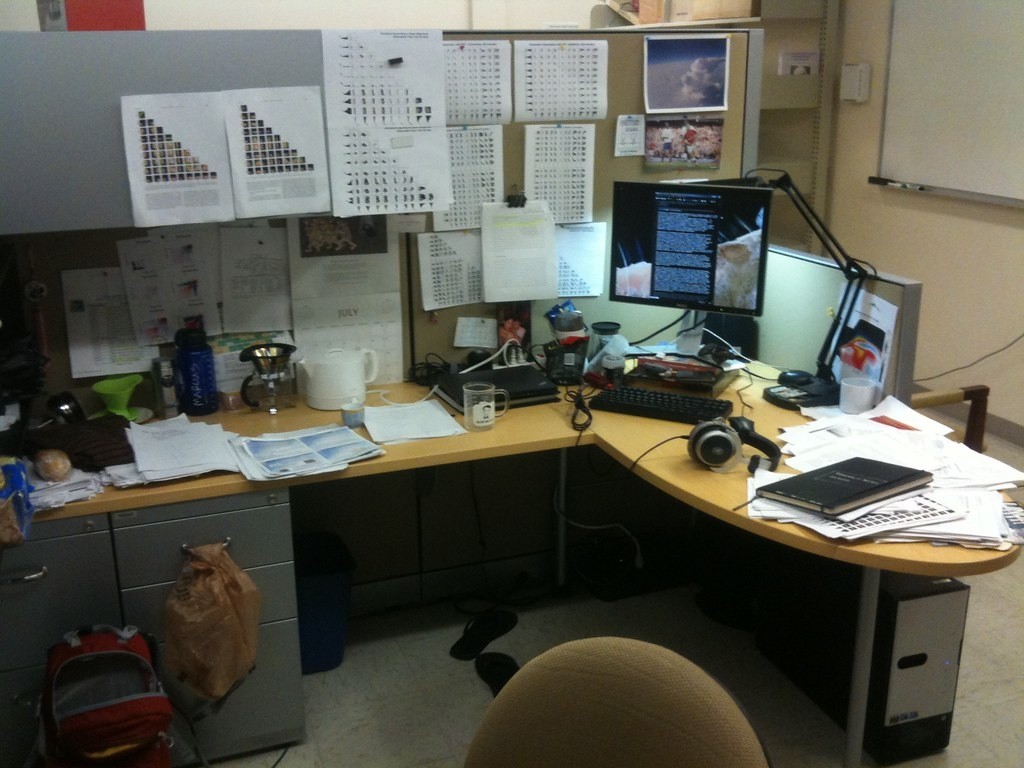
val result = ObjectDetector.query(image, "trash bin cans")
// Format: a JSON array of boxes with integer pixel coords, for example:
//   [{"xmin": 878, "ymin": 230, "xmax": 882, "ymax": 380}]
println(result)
[{"xmin": 293, "ymin": 531, "xmax": 357, "ymax": 675}]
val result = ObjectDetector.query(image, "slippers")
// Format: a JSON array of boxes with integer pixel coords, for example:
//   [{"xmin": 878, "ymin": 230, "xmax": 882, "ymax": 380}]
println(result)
[
  {"xmin": 449, "ymin": 607, "xmax": 518, "ymax": 661},
  {"xmin": 475, "ymin": 653, "xmax": 520, "ymax": 698}
]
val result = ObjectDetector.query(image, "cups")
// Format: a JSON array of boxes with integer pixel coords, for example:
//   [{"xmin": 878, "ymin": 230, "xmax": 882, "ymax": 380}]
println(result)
[
  {"xmin": 463, "ymin": 381, "xmax": 510, "ymax": 431},
  {"xmin": 92, "ymin": 374, "xmax": 143, "ymax": 422},
  {"xmin": 338, "ymin": 403, "xmax": 364, "ymax": 432},
  {"xmin": 839, "ymin": 377, "xmax": 882, "ymax": 414}
]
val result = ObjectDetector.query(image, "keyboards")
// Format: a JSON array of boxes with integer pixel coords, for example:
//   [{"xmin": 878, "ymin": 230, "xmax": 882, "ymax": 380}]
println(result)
[{"xmin": 588, "ymin": 384, "xmax": 734, "ymax": 424}]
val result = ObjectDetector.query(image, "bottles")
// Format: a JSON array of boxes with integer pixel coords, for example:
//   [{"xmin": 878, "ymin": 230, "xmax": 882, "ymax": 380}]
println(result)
[
  {"xmin": 35, "ymin": 384, "xmax": 103, "ymax": 431},
  {"xmin": 151, "ymin": 329, "xmax": 219, "ymax": 418},
  {"xmin": 556, "ymin": 311, "xmax": 625, "ymax": 390}
]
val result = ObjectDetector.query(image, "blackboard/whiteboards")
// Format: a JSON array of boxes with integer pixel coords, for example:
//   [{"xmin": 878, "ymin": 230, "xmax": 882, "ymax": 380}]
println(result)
[{"xmin": 878, "ymin": 0, "xmax": 1024, "ymax": 209}]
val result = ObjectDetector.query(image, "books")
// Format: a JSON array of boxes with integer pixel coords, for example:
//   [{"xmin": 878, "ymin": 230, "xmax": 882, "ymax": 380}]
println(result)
[{"xmin": 755, "ymin": 457, "xmax": 934, "ymax": 516}]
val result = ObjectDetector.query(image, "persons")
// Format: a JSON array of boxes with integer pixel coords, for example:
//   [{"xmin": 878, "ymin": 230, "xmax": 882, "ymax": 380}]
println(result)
[
  {"xmin": 660, "ymin": 121, "xmax": 697, "ymax": 163},
  {"xmin": 498, "ymin": 315, "xmax": 525, "ymax": 346}
]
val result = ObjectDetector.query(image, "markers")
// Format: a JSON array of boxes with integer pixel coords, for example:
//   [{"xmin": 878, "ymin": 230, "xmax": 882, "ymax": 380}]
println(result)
[
  {"xmin": 887, "ymin": 182, "xmax": 906, "ymax": 188},
  {"xmin": 906, "ymin": 183, "xmax": 924, "ymax": 191}
]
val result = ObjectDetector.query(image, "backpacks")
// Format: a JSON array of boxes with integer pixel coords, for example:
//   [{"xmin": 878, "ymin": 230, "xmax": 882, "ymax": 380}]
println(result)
[{"xmin": 25, "ymin": 623, "xmax": 173, "ymax": 768}]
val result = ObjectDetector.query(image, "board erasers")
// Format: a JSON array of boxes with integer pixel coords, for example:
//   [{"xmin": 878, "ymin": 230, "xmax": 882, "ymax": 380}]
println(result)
[{"xmin": 868, "ymin": 176, "xmax": 892, "ymax": 185}]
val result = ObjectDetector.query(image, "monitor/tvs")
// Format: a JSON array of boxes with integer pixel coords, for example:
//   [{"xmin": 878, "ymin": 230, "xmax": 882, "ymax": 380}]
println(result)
[{"xmin": 610, "ymin": 182, "xmax": 774, "ymax": 316}]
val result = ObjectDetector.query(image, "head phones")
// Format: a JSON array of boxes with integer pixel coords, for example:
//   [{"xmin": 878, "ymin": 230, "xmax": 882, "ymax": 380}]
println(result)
[{"xmin": 688, "ymin": 420, "xmax": 782, "ymax": 473}]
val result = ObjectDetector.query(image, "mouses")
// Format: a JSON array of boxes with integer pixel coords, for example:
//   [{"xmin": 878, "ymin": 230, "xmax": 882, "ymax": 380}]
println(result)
[{"xmin": 778, "ymin": 370, "xmax": 812, "ymax": 386}]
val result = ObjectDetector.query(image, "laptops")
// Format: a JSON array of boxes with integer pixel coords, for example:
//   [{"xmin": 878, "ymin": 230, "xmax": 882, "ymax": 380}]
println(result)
[{"xmin": 438, "ymin": 366, "xmax": 557, "ymax": 407}]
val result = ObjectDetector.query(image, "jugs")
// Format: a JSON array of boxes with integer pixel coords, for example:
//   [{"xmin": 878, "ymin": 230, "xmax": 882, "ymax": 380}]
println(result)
[{"xmin": 240, "ymin": 342, "xmax": 300, "ymax": 413}]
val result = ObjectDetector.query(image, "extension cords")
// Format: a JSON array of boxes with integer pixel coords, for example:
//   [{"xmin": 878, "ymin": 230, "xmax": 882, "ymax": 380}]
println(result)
[{"xmin": 493, "ymin": 353, "xmax": 548, "ymax": 370}]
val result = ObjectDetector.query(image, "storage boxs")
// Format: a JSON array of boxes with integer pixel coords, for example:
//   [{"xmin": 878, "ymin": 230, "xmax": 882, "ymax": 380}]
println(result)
[{"xmin": 639, "ymin": 0, "xmax": 761, "ymax": 24}]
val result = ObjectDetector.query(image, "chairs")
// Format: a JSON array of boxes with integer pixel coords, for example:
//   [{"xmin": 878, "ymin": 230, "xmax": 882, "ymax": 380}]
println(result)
[{"xmin": 465, "ymin": 637, "xmax": 771, "ymax": 768}]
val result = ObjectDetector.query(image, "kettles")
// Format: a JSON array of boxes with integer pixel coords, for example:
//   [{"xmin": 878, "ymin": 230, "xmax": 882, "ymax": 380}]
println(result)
[{"xmin": 298, "ymin": 347, "xmax": 379, "ymax": 410}]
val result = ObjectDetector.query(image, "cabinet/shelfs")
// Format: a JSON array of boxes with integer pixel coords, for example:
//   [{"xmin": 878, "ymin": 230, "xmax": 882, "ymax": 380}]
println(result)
[
  {"xmin": 1, "ymin": 486, "xmax": 308, "ymax": 768},
  {"xmin": 642, "ymin": 0, "xmax": 840, "ymax": 257}
]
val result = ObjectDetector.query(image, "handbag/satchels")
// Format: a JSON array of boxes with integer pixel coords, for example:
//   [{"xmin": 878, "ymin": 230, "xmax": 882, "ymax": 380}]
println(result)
[{"xmin": 0, "ymin": 392, "xmax": 135, "ymax": 472}]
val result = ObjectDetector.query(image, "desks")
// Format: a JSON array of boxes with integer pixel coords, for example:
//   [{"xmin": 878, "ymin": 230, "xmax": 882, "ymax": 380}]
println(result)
[{"xmin": 0, "ymin": 356, "xmax": 1024, "ymax": 768}]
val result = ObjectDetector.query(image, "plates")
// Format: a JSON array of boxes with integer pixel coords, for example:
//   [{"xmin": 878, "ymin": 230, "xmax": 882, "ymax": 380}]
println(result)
[{"xmin": 88, "ymin": 406, "xmax": 154, "ymax": 424}]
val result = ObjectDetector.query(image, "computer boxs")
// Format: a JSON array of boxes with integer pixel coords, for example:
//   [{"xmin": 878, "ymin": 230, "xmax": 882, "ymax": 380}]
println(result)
[{"xmin": 777, "ymin": 553, "xmax": 971, "ymax": 766}]
